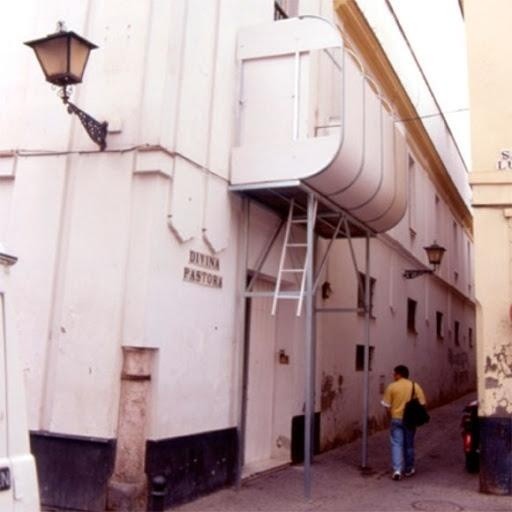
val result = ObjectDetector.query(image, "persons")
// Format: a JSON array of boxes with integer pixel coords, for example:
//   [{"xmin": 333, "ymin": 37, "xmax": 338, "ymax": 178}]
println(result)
[{"xmin": 378, "ymin": 363, "xmax": 426, "ymax": 480}]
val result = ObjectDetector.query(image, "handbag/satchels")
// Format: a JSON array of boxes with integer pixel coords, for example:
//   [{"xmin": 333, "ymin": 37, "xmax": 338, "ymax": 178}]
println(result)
[{"xmin": 403, "ymin": 399, "xmax": 430, "ymax": 427}]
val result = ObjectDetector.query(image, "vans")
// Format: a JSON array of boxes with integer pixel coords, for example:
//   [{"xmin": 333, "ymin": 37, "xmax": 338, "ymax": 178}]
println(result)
[{"xmin": 0, "ymin": 251, "xmax": 42, "ymax": 511}]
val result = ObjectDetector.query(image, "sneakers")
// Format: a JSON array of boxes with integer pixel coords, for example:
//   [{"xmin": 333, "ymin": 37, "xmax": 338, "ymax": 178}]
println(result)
[
  {"xmin": 405, "ymin": 468, "xmax": 415, "ymax": 476},
  {"xmin": 392, "ymin": 471, "xmax": 401, "ymax": 480}
]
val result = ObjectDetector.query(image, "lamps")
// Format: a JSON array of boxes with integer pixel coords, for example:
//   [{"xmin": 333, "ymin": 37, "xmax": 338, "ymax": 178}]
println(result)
[
  {"xmin": 398, "ymin": 241, "xmax": 446, "ymax": 279},
  {"xmin": 26, "ymin": 17, "xmax": 112, "ymax": 154}
]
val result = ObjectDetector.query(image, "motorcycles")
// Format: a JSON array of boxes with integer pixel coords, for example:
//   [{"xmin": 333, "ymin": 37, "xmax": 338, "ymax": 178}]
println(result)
[{"xmin": 459, "ymin": 399, "xmax": 479, "ymax": 474}]
